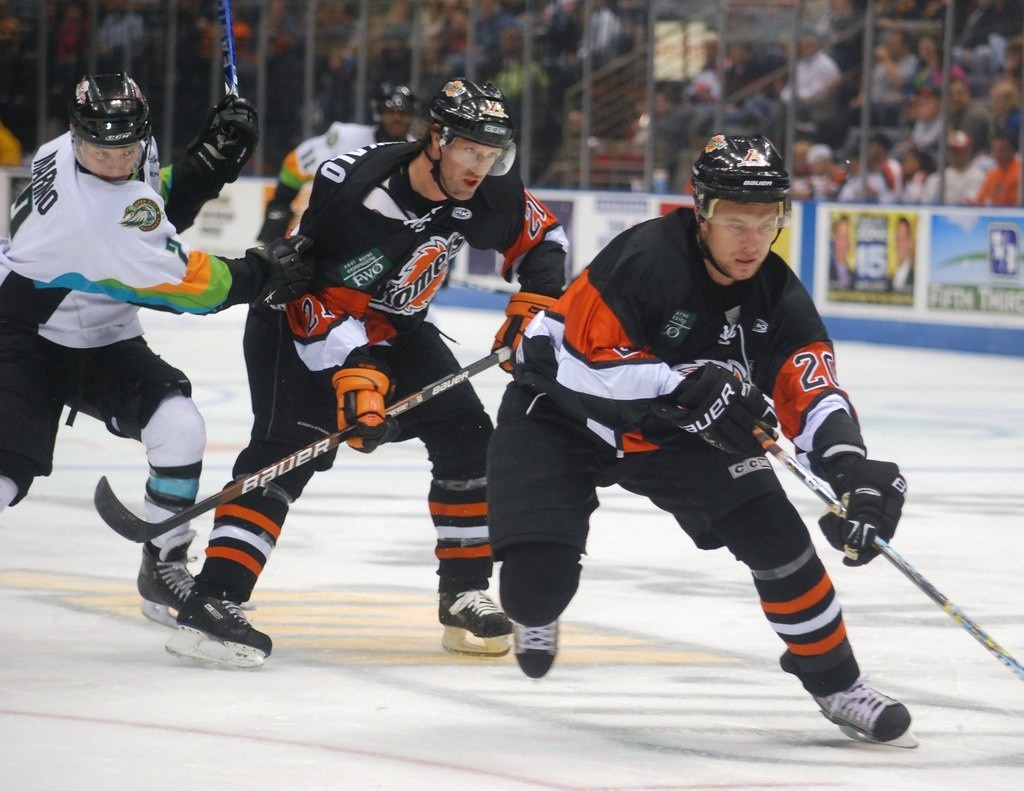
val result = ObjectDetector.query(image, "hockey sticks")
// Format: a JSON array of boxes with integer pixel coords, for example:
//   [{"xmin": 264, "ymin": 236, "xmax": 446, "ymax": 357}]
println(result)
[
  {"xmin": 94, "ymin": 346, "xmax": 512, "ymax": 544},
  {"xmin": 752, "ymin": 426, "xmax": 1024, "ymax": 683},
  {"xmin": 217, "ymin": 0, "xmax": 239, "ymax": 98}
]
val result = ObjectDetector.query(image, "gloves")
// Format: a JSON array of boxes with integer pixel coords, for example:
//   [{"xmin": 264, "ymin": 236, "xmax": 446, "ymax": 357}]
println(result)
[
  {"xmin": 653, "ymin": 363, "xmax": 779, "ymax": 455},
  {"xmin": 818, "ymin": 453, "xmax": 906, "ymax": 567},
  {"xmin": 187, "ymin": 93, "xmax": 259, "ymax": 190},
  {"xmin": 331, "ymin": 363, "xmax": 400, "ymax": 455},
  {"xmin": 491, "ymin": 294, "xmax": 560, "ymax": 373},
  {"xmin": 247, "ymin": 233, "xmax": 316, "ymax": 315}
]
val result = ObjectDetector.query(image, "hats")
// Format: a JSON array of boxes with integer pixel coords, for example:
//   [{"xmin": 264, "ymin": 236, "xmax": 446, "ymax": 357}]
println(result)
[
  {"xmin": 807, "ymin": 142, "xmax": 835, "ymax": 165},
  {"xmin": 949, "ymin": 133, "xmax": 971, "ymax": 155}
]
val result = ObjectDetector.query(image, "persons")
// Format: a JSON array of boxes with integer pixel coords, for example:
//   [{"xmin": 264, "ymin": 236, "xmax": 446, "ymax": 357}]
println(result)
[
  {"xmin": 485, "ymin": 133, "xmax": 920, "ymax": 750},
  {"xmin": 0, "ymin": 71, "xmax": 319, "ymax": 628},
  {"xmin": 655, "ymin": 0, "xmax": 1024, "ymax": 204},
  {"xmin": 166, "ymin": 76, "xmax": 569, "ymax": 668},
  {"xmin": 255, "ymin": 83, "xmax": 418, "ymax": 246},
  {"xmin": 0, "ymin": 0, "xmax": 633, "ymax": 194}
]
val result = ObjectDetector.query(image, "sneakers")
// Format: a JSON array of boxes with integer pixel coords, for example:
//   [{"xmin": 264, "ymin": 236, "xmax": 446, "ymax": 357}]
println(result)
[
  {"xmin": 137, "ymin": 530, "xmax": 199, "ymax": 629},
  {"xmin": 813, "ymin": 682, "xmax": 919, "ymax": 750},
  {"xmin": 164, "ymin": 584, "xmax": 273, "ymax": 668},
  {"xmin": 515, "ymin": 618, "xmax": 560, "ymax": 679},
  {"xmin": 438, "ymin": 588, "xmax": 514, "ymax": 655}
]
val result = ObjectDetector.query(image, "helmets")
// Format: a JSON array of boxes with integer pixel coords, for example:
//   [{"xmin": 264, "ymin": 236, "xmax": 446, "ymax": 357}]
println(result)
[
  {"xmin": 71, "ymin": 72, "xmax": 152, "ymax": 177},
  {"xmin": 369, "ymin": 79, "xmax": 414, "ymax": 121},
  {"xmin": 431, "ymin": 77, "xmax": 517, "ymax": 176},
  {"xmin": 690, "ymin": 134, "xmax": 795, "ymax": 229}
]
